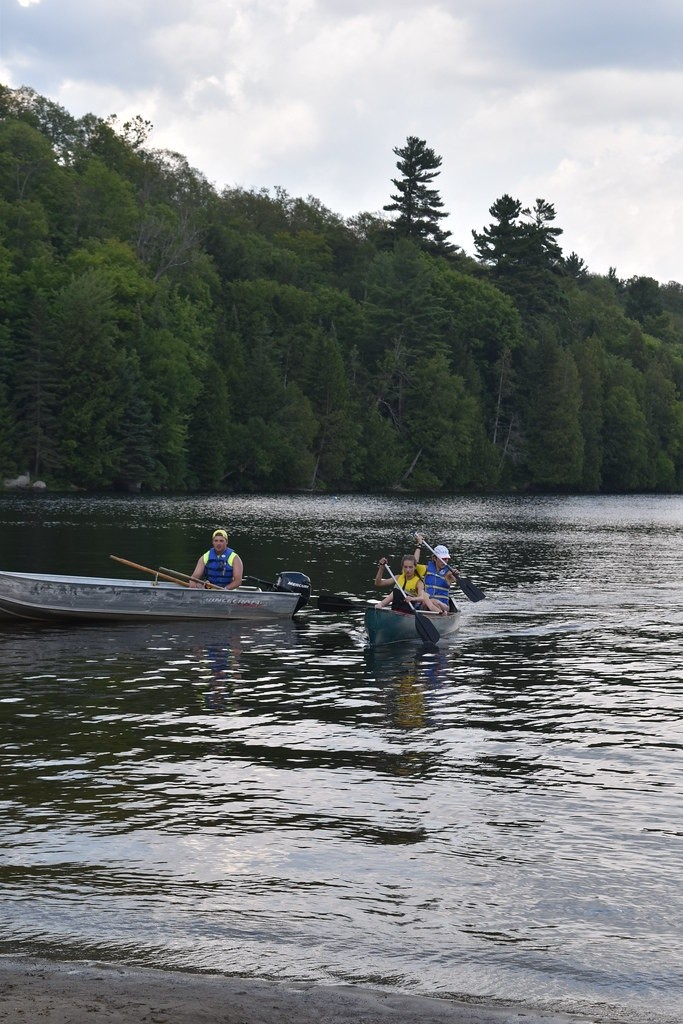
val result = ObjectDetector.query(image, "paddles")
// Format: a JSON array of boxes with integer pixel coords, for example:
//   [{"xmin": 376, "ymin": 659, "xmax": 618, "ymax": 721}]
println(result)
[
  {"xmin": 414, "ymin": 531, "xmax": 486, "ymax": 603},
  {"xmin": 384, "ymin": 559, "xmax": 440, "ymax": 645},
  {"xmin": 315, "ymin": 593, "xmax": 445, "ymax": 616}
]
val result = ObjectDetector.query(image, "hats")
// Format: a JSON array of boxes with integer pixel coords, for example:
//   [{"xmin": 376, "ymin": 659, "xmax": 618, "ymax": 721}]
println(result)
[
  {"xmin": 434, "ymin": 545, "xmax": 451, "ymax": 558},
  {"xmin": 212, "ymin": 530, "xmax": 227, "ymax": 540}
]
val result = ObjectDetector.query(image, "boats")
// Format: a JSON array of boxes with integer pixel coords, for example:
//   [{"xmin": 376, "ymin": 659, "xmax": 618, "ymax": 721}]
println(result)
[
  {"xmin": 364, "ymin": 594, "xmax": 462, "ymax": 648},
  {"xmin": 0, "ymin": 570, "xmax": 312, "ymax": 619}
]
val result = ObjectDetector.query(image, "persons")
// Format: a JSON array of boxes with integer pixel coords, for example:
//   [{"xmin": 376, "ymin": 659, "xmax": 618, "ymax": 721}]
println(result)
[
  {"xmin": 367, "ymin": 531, "xmax": 461, "ymax": 617},
  {"xmin": 189, "ymin": 529, "xmax": 243, "ymax": 590}
]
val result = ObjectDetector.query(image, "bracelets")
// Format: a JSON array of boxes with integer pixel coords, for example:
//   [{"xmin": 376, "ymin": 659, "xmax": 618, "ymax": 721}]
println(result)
[{"xmin": 416, "ymin": 542, "xmax": 422, "ymax": 548}]
